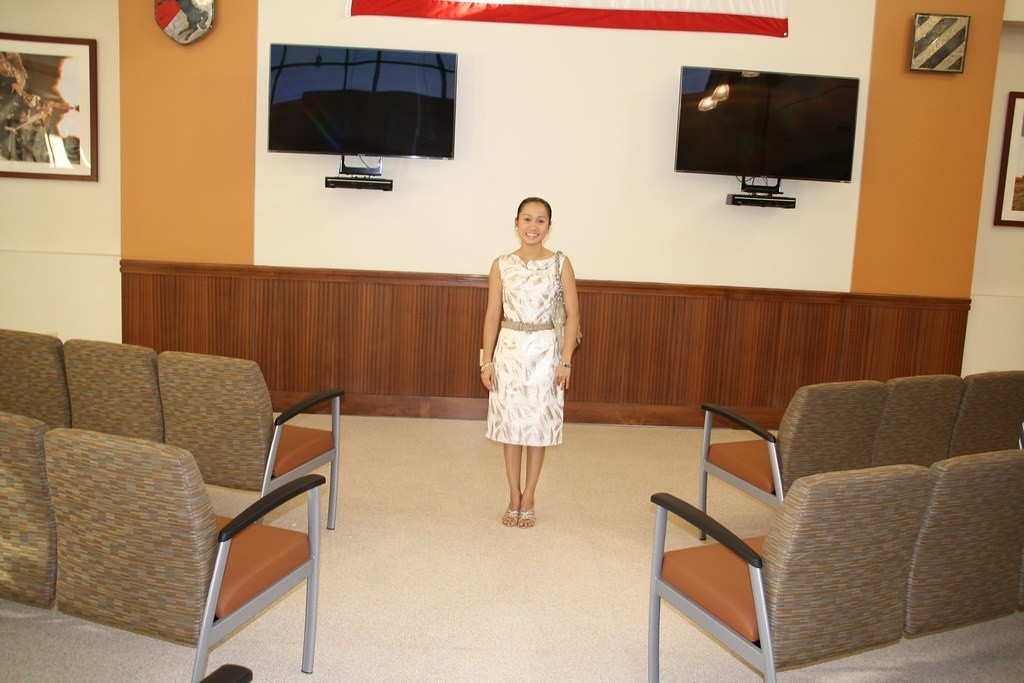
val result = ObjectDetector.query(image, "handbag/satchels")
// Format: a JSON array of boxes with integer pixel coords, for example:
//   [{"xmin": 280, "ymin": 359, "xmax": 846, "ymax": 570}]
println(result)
[{"xmin": 548, "ymin": 251, "xmax": 582, "ymax": 354}]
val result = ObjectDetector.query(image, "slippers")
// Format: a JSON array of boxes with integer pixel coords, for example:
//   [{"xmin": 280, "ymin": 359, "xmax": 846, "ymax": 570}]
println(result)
[
  {"xmin": 518, "ymin": 507, "xmax": 535, "ymax": 529},
  {"xmin": 503, "ymin": 506, "xmax": 520, "ymax": 527}
]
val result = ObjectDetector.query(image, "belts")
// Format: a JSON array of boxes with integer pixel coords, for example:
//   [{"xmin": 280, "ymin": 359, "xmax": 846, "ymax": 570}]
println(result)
[{"xmin": 501, "ymin": 318, "xmax": 555, "ymax": 334}]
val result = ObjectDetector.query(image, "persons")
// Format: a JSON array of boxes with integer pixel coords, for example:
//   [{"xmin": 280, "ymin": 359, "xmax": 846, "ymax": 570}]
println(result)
[{"xmin": 481, "ymin": 197, "xmax": 580, "ymax": 529}]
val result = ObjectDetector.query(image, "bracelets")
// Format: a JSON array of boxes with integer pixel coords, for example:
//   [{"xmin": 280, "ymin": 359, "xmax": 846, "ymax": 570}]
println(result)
[
  {"xmin": 481, "ymin": 363, "xmax": 494, "ymax": 372},
  {"xmin": 560, "ymin": 361, "xmax": 572, "ymax": 368}
]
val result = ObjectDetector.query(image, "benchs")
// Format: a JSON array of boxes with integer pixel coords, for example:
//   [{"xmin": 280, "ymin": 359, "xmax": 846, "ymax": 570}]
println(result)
[
  {"xmin": 693, "ymin": 369, "xmax": 1023, "ymax": 538},
  {"xmin": 0, "ymin": 408, "xmax": 327, "ymax": 681},
  {"xmin": 647, "ymin": 451, "xmax": 1023, "ymax": 682},
  {"xmin": 1, "ymin": 327, "xmax": 344, "ymax": 535}
]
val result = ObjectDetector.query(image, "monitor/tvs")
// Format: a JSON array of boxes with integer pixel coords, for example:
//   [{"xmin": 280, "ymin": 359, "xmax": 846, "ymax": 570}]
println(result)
[
  {"xmin": 266, "ymin": 43, "xmax": 457, "ymax": 177},
  {"xmin": 674, "ymin": 65, "xmax": 860, "ymax": 196}
]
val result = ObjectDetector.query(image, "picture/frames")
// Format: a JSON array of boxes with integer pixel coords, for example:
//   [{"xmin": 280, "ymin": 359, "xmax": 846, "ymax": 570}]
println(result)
[
  {"xmin": 908, "ymin": 12, "xmax": 970, "ymax": 73},
  {"xmin": 0, "ymin": 32, "xmax": 99, "ymax": 181},
  {"xmin": 993, "ymin": 92, "xmax": 1024, "ymax": 227}
]
